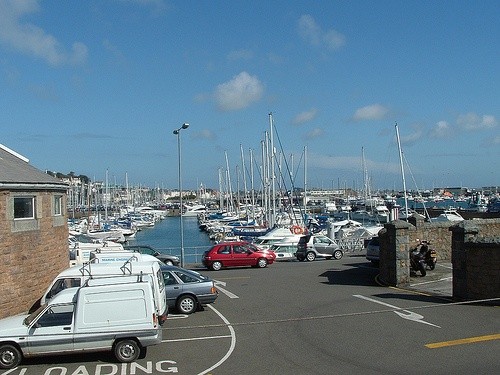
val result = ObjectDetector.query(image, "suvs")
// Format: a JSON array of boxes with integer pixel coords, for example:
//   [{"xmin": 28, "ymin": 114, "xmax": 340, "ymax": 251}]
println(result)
[
  {"xmin": 0, "ymin": 286, "xmax": 163, "ymax": 368},
  {"xmin": 364, "ymin": 235, "xmax": 383, "ymax": 266},
  {"xmin": 38, "ymin": 258, "xmax": 169, "ymax": 325}
]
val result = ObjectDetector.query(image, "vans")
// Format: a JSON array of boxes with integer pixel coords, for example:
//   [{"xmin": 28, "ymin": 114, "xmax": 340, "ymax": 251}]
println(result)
[{"xmin": 296, "ymin": 234, "xmax": 344, "ymax": 261}]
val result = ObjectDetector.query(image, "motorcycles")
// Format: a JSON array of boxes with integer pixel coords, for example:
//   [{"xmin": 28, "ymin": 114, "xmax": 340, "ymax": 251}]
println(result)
[
  {"xmin": 416, "ymin": 237, "xmax": 436, "ymax": 270},
  {"xmin": 409, "ymin": 248, "xmax": 427, "ymax": 277}
]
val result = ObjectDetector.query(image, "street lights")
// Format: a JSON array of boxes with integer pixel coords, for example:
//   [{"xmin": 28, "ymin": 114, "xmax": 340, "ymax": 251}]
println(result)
[{"xmin": 173, "ymin": 123, "xmax": 191, "ymax": 267}]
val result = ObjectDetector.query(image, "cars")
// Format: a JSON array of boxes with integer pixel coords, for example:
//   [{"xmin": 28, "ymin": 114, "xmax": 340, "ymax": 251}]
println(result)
[
  {"xmin": 159, "ymin": 265, "xmax": 217, "ymax": 315},
  {"xmin": 258, "ymin": 235, "xmax": 306, "ymax": 262},
  {"xmin": 203, "ymin": 241, "xmax": 276, "ymax": 270},
  {"xmin": 68, "ymin": 241, "xmax": 180, "ymax": 268}
]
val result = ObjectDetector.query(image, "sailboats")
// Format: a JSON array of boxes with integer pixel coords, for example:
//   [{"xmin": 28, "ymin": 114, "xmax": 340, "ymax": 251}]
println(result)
[{"xmin": 68, "ymin": 111, "xmax": 500, "ymax": 252}]
